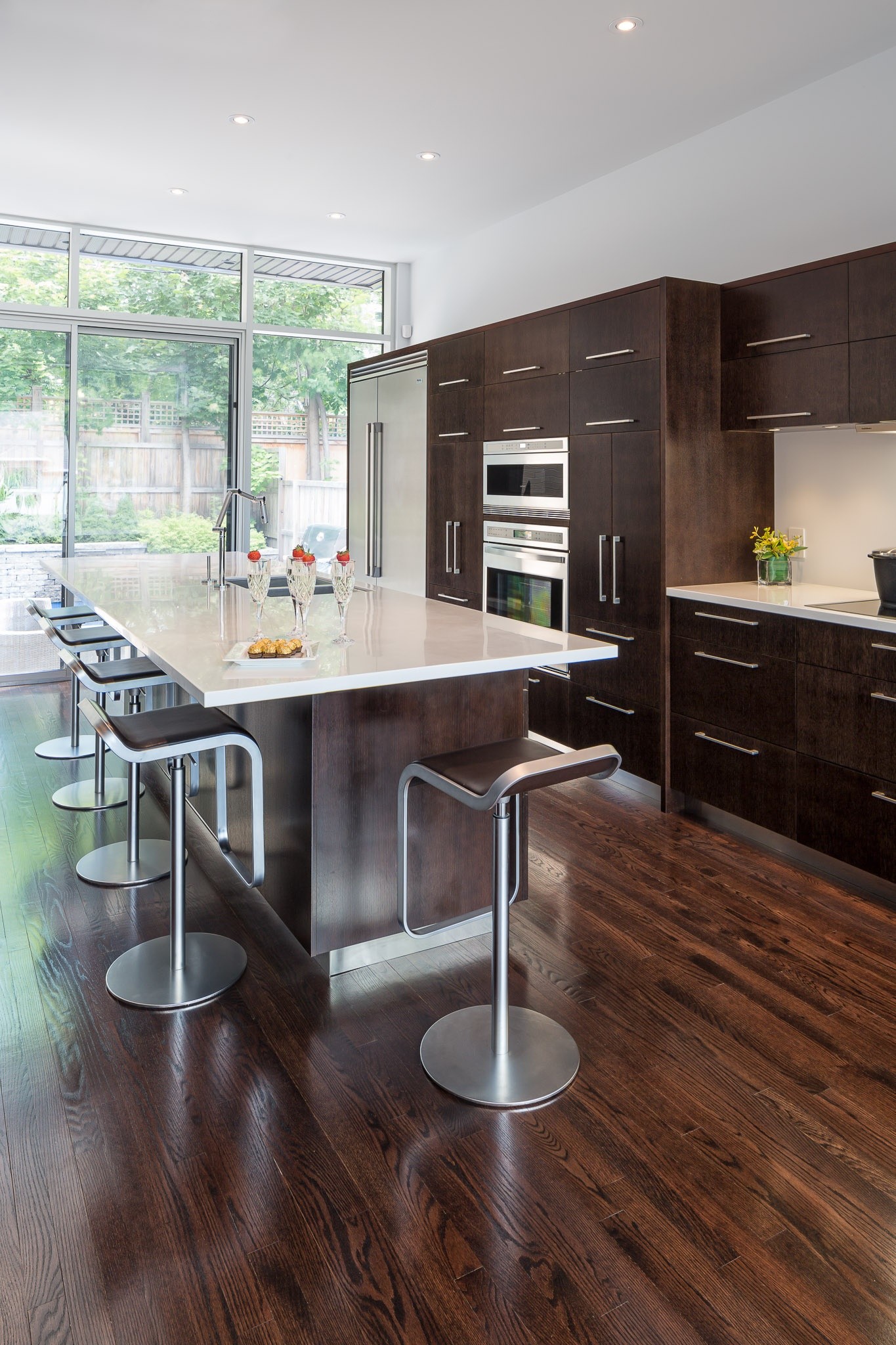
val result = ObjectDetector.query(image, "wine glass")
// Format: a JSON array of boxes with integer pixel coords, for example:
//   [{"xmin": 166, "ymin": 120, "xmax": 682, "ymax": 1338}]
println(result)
[
  {"xmin": 248, "ymin": 559, "xmax": 271, "ymax": 641},
  {"xmin": 330, "ymin": 560, "xmax": 355, "ymax": 645},
  {"xmin": 292, "ymin": 560, "xmax": 317, "ymax": 640},
  {"xmin": 287, "ymin": 556, "xmax": 308, "ymax": 636}
]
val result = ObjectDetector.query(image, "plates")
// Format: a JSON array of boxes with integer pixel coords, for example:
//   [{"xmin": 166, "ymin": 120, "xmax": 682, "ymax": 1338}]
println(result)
[{"xmin": 222, "ymin": 641, "xmax": 322, "ymax": 665}]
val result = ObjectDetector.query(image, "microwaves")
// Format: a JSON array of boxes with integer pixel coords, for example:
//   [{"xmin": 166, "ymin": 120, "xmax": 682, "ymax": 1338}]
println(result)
[{"xmin": 483, "ymin": 437, "xmax": 568, "ymax": 510}]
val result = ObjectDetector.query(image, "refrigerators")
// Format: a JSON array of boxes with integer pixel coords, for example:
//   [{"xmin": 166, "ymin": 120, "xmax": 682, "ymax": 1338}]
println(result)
[{"xmin": 349, "ymin": 365, "xmax": 427, "ymax": 597}]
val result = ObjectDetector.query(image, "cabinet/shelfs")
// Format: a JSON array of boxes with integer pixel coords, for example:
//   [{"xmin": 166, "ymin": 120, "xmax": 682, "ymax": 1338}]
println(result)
[{"xmin": 347, "ymin": 241, "xmax": 895, "ymax": 906}]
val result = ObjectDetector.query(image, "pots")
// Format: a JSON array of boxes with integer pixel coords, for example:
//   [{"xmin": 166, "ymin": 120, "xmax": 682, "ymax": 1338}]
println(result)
[{"xmin": 867, "ymin": 548, "xmax": 895, "ymax": 609}]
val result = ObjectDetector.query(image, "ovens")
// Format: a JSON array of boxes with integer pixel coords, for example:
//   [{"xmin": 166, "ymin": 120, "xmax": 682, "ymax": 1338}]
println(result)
[{"xmin": 482, "ymin": 542, "xmax": 568, "ymax": 677}]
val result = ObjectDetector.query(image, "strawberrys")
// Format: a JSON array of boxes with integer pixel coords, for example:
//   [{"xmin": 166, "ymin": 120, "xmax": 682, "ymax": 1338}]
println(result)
[
  {"xmin": 247, "ymin": 548, "xmax": 261, "ymax": 563},
  {"xmin": 336, "ymin": 550, "xmax": 351, "ymax": 567},
  {"xmin": 293, "ymin": 544, "xmax": 304, "ymax": 561},
  {"xmin": 299, "ymin": 547, "xmax": 315, "ymax": 568}
]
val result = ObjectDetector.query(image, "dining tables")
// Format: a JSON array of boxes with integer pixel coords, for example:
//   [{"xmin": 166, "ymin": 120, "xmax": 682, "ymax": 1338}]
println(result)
[{"xmin": 40, "ymin": 553, "xmax": 617, "ymax": 981}]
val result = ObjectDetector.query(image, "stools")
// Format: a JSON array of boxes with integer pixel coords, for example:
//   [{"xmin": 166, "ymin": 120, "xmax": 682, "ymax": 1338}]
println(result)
[
  {"xmin": 24, "ymin": 598, "xmax": 105, "ymax": 758},
  {"xmin": 75, "ymin": 697, "xmax": 266, "ymax": 1010},
  {"xmin": 395, "ymin": 740, "xmax": 621, "ymax": 1107},
  {"xmin": 38, "ymin": 617, "xmax": 134, "ymax": 810},
  {"xmin": 57, "ymin": 647, "xmax": 176, "ymax": 886}
]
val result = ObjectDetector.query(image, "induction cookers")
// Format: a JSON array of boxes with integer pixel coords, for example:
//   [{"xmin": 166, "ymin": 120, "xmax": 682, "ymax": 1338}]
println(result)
[{"xmin": 804, "ymin": 598, "xmax": 896, "ymax": 620}]
